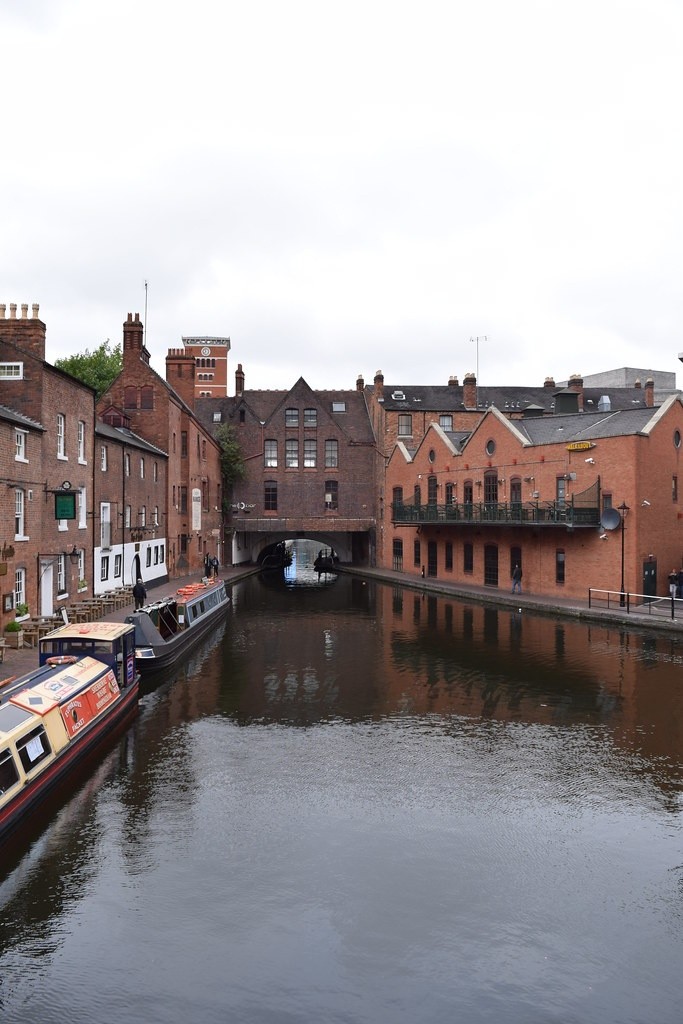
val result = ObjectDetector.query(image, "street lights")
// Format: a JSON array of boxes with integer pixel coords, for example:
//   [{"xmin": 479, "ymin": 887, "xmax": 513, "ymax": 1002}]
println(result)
[
  {"xmin": 617, "ymin": 499, "xmax": 630, "ymax": 607},
  {"xmin": 166, "ymin": 533, "xmax": 193, "ymax": 575},
  {"xmin": 36, "ymin": 544, "xmax": 80, "ymax": 618}
]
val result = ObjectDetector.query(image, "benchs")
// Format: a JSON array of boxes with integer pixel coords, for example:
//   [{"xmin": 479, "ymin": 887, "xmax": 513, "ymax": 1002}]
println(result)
[{"xmin": 0, "ymin": 590, "xmax": 135, "ymax": 662}]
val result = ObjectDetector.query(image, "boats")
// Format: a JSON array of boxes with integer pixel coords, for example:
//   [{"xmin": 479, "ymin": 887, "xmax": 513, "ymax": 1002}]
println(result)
[
  {"xmin": 0, "ymin": 621, "xmax": 141, "ymax": 851},
  {"xmin": 313, "ymin": 548, "xmax": 338, "ymax": 571},
  {"xmin": 266, "ymin": 540, "xmax": 292, "ymax": 568},
  {"xmin": 124, "ymin": 576, "xmax": 231, "ymax": 671}
]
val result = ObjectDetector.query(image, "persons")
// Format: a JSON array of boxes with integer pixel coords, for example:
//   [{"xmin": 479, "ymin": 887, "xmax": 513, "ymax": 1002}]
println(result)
[
  {"xmin": 132, "ymin": 579, "xmax": 147, "ymax": 609},
  {"xmin": 211, "ymin": 555, "xmax": 219, "ymax": 578},
  {"xmin": 203, "ymin": 552, "xmax": 215, "ymax": 578},
  {"xmin": 668, "ymin": 568, "xmax": 683, "ymax": 598},
  {"xmin": 510, "ymin": 560, "xmax": 523, "ymax": 595}
]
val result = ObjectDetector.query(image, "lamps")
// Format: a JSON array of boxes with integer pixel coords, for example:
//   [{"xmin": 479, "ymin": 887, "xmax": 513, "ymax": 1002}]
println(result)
[{"xmin": 38, "ymin": 545, "xmax": 80, "ymax": 565}]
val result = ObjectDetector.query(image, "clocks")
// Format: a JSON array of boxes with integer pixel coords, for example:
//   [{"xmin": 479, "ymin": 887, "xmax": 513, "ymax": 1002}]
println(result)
[{"xmin": 200, "ymin": 347, "xmax": 210, "ymax": 356}]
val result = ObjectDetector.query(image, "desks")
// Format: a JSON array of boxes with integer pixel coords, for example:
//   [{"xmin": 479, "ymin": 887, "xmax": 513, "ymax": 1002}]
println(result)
[
  {"xmin": 116, "ymin": 587, "xmax": 134, "ymax": 603},
  {"xmin": 20, "ymin": 622, "xmax": 40, "ymax": 646},
  {"xmin": 83, "ymin": 598, "xmax": 103, "ymax": 618},
  {"xmin": 105, "ymin": 589, "xmax": 126, "ymax": 607},
  {"xmin": 96, "ymin": 593, "xmax": 117, "ymax": 611},
  {"xmin": 33, "ymin": 616, "xmax": 54, "ymax": 631},
  {"xmin": 72, "ymin": 602, "xmax": 93, "ymax": 623},
  {"xmin": 67, "ymin": 608, "xmax": 79, "ymax": 623}
]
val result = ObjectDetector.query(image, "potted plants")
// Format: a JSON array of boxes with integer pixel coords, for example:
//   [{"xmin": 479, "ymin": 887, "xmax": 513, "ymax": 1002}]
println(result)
[
  {"xmin": 15, "ymin": 602, "xmax": 30, "ymax": 622},
  {"xmin": 4, "ymin": 622, "xmax": 25, "ymax": 650},
  {"xmin": 76, "ymin": 579, "xmax": 88, "ymax": 594}
]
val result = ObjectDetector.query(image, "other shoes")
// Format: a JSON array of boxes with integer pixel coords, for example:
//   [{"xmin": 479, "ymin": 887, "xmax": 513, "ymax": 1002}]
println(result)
[
  {"xmin": 510, "ymin": 591, "xmax": 514, "ymax": 594},
  {"xmin": 517, "ymin": 592, "xmax": 521, "ymax": 595}
]
